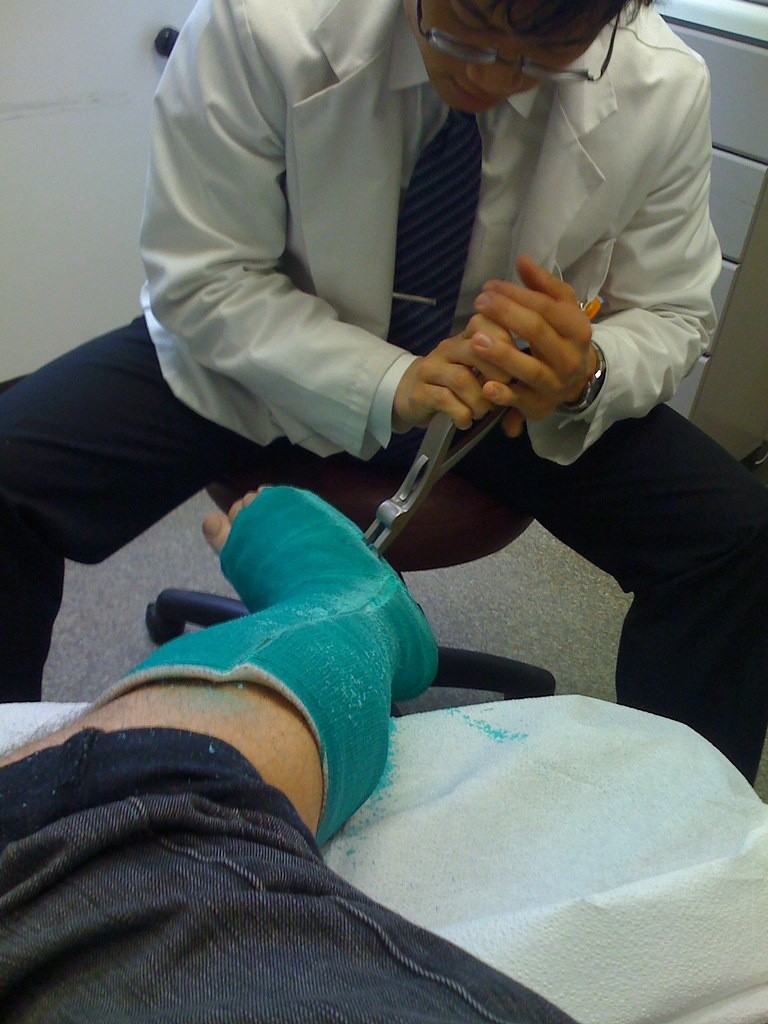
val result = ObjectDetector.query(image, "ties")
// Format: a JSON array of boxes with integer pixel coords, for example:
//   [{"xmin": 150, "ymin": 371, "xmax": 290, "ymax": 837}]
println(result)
[{"xmin": 386, "ymin": 109, "xmax": 483, "ymax": 357}]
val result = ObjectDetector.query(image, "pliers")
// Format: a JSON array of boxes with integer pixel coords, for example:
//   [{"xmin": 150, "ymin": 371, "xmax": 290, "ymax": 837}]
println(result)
[{"xmin": 363, "ymin": 343, "xmax": 534, "ymax": 556}]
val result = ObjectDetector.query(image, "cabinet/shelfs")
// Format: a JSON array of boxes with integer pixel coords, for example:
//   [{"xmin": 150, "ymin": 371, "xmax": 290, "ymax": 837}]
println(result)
[{"xmin": 654, "ymin": 23, "xmax": 768, "ymax": 421}]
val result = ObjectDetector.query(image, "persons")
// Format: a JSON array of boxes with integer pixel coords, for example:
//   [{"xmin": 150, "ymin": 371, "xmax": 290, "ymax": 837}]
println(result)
[
  {"xmin": 2, "ymin": 0, "xmax": 767, "ymax": 787},
  {"xmin": 0, "ymin": 487, "xmax": 581, "ymax": 1024}
]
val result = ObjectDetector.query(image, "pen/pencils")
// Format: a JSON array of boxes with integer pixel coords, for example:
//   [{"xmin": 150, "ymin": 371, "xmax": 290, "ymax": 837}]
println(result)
[{"xmin": 582, "ymin": 294, "xmax": 604, "ymax": 320}]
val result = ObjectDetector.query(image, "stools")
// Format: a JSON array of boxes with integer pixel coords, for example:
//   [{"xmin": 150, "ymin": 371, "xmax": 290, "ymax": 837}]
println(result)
[{"xmin": 146, "ymin": 483, "xmax": 556, "ymax": 717}]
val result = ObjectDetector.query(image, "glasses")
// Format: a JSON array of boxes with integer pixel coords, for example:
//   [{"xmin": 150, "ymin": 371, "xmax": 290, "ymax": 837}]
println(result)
[{"xmin": 416, "ymin": 0, "xmax": 625, "ymax": 83}]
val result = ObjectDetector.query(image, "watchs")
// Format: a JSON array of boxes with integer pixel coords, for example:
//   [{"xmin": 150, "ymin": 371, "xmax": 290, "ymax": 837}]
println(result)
[{"xmin": 560, "ymin": 340, "xmax": 608, "ymax": 410}]
